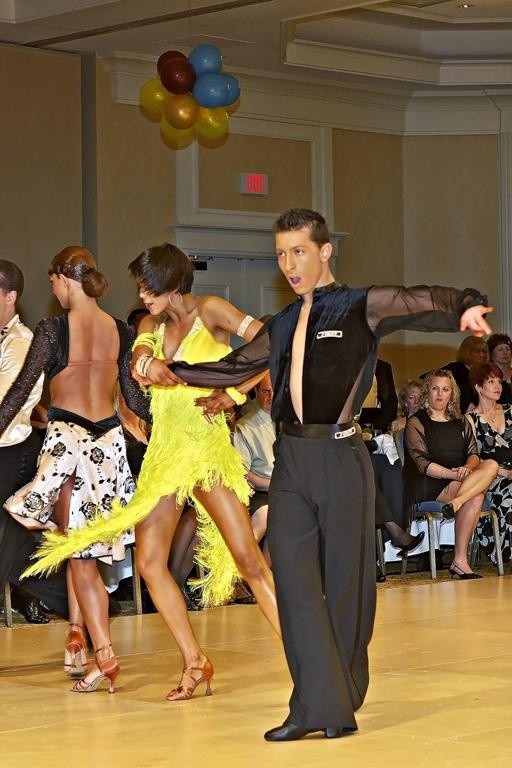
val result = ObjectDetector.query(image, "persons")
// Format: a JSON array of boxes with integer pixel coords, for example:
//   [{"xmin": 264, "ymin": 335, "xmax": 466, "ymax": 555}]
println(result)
[
  {"xmin": 356, "ymin": 335, "xmax": 511, "ymax": 581},
  {"xmin": 169, "ymin": 370, "xmax": 285, "ymax": 612},
  {"xmin": 0, "ymin": 245, "xmax": 151, "ymax": 692},
  {"xmin": 1, "ymin": 258, "xmax": 44, "ymax": 600},
  {"xmin": 129, "ymin": 208, "xmax": 495, "ymax": 742},
  {"xmin": 128, "ymin": 240, "xmax": 286, "ymax": 703}
]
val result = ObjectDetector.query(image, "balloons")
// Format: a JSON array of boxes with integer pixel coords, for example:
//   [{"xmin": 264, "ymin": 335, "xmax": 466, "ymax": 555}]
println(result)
[{"xmin": 139, "ymin": 43, "xmax": 241, "ymax": 149}]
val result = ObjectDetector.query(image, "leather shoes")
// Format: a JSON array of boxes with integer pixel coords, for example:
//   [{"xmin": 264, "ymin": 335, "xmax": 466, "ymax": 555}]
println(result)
[
  {"xmin": 264, "ymin": 720, "xmax": 343, "ymax": 740},
  {"xmin": 38, "ymin": 599, "xmax": 53, "ymax": 613},
  {"xmin": 11, "ymin": 594, "xmax": 49, "ymax": 623},
  {"xmin": 236, "ymin": 581, "xmax": 256, "ymax": 603}
]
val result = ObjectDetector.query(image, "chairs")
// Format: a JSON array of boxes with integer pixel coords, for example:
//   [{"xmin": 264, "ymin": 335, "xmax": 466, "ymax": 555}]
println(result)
[
  {"xmin": 392, "ymin": 428, "xmax": 505, "ymax": 579},
  {"xmin": 129, "ymin": 521, "xmax": 210, "ymax": 615},
  {"xmin": 4, "ymin": 577, "xmax": 12, "ymax": 627},
  {"xmin": 374, "ymin": 527, "xmax": 388, "ymax": 579}
]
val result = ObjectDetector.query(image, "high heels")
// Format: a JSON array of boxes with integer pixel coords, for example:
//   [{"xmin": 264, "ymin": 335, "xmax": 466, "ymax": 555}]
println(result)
[
  {"xmin": 440, "ymin": 503, "xmax": 454, "ymax": 523},
  {"xmin": 166, "ymin": 656, "xmax": 213, "ymax": 699},
  {"xmin": 64, "ymin": 623, "xmax": 87, "ymax": 679},
  {"xmin": 71, "ymin": 643, "xmax": 119, "ymax": 693},
  {"xmin": 376, "ymin": 565, "xmax": 386, "ymax": 581},
  {"xmin": 397, "ymin": 530, "xmax": 425, "ymax": 560},
  {"xmin": 450, "ymin": 560, "xmax": 484, "ymax": 578}
]
